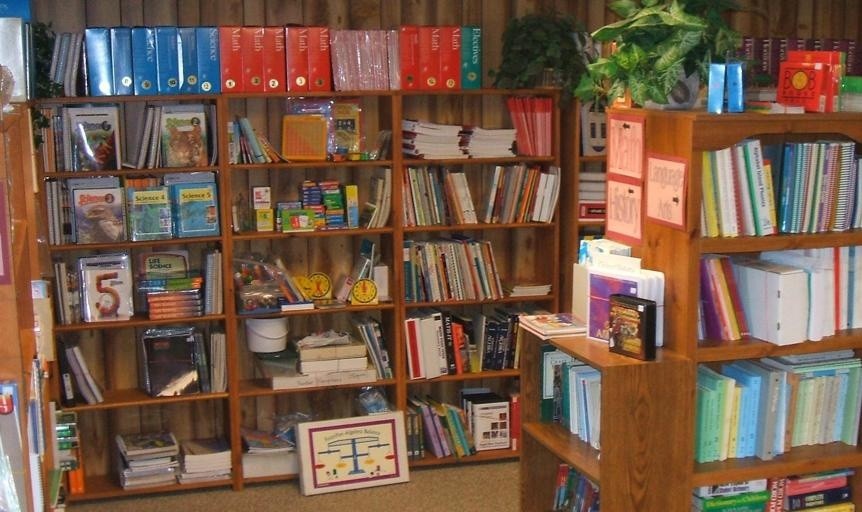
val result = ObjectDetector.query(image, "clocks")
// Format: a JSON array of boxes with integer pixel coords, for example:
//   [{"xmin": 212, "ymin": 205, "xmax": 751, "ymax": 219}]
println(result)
[
  {"xmin": 308, "ymin": 272, "xmax": 333, "ymax": 300},
  {"xmin": 351, "ymin": 279, "xmax": 379, "ymax": 305}
]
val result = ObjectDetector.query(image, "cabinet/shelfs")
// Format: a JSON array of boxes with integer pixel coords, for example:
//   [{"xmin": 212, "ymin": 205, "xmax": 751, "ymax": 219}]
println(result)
[
  {"xmin": 518, "ymin": 106, "xmax": 862, "ymax": 512},
  {"xmin": 36, "ymin": 88, "xmax": 561, "ymax": 512},
  {"xmin": 561, "ymin": 97, "xmax": 607, "ymax": 314},
  {"xmin": 0, "ymin": 98, "xmax": 67, "ymax": 512}
]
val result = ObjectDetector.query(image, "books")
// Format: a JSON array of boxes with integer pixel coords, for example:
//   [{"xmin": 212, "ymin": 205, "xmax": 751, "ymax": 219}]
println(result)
[
  {"xmin": 553, "ymin": 463, "xmax": 599, "ymax": 512},
  {"xmin": 406, "ymin": 388, "xmax": 520, "ymax": 459},
  {"xmin": 53, "ymin": 248, "xmax": 222, "ymax": 325},
  {"xmin": 692, "ymin": 135, "xmax": 862, "ymax": 512},
  {"xmin": 237, "ymin": 238, "xmax": 393, "ymax": 389},
  {"xmin": 578, "ymin": 171, "xmax": 608, "ymax": 225},
  {"xmin": 537, "ymin": 343, "xmax": 600, "ymax": 449},
  {"xmin": 406, "ymin": 305, "xmax": 550, "ymax": 379},
  {"xmin": 228, "ymin": 113, "xmax": 392, "ymax": 230},
  {"xmin": 404, "ymin": 164, "xmax": 560, "ymax": 227},
  {"xmin": 517, "ymin": 238, "xmax": 666, "ymax": 361},
  {"xmin": 402, "ymin": 97, "xmax": 552, "ymax": 158},
  {"xmin": 45, "ymin": 172, "xmax": 220, "ymax": 247},
  {"xmin": 732, "ymin": 34, "xmax": 862, "ymax": 115},
  {"xmin": 56, "ymin": 325, "xmax": 227, "ymax": 406},
  {"xmin": 241, "ymin": 426, "xmax": 300, "ymax": 477},
  {"xmin": 30, "ymin": 358, "xmax": 232, "ymax": 512},
  {"xmin": 404, "ymin": 237, "xmax": 551, "ymax": 302},
  {"xmin": 41, "ymin": 104, "xmax": 217, "ymax": 173}
]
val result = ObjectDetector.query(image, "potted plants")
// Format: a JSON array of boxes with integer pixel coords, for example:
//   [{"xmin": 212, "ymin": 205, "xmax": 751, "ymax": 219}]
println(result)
[
  {"xmin": 487, "ymin": 6, "xmax": 598, "ymax": 98},
  {"xmin": 574, "ymin": 0, "xmax": 750, "ymax": 111}
]
